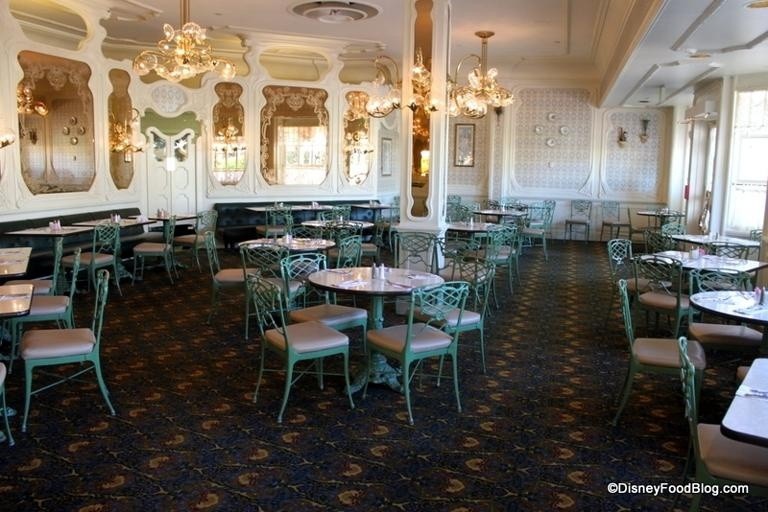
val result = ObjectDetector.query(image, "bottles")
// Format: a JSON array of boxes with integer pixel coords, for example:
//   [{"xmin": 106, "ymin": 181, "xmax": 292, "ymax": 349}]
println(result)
[
  {"xmin": 753, "ymin": 285, "xmax": 768, "ymax": 307},
  {"xmin": 688, "ymin": 245, "xmax": 707, "ymax": 258},
  {"xmin": 53, "ymin": 218, "xmax": 61, "ymax": 231},
  {"xmin": 110, "ymin": 210, "xmax": 119, "ymax": 224},
  {"xmin": 371, "ymin": 263, "xmax": 385, "ymax": 280}
]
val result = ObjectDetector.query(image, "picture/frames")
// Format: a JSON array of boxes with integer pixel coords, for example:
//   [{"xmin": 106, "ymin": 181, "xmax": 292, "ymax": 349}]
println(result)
[
  {"xmin": 380, "ymin": 137, "xmax": 393, "ymax": 176},
  {"xmin": 454, "ymin": 123, "xmax": 476, "ymax": 167}
]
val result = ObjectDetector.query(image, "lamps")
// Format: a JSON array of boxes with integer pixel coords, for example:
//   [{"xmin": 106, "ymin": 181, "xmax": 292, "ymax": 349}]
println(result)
[
  {"xmin": 361, "ymin": 46, "xmax": 516, "ymax": 119},
  {"xmin": 111, "ymin": 107, "xmax": 146, "ymax": 163},
  {"xmin": 129, "ymin": 0, "xmax": 240, "ymax": 82}
]
